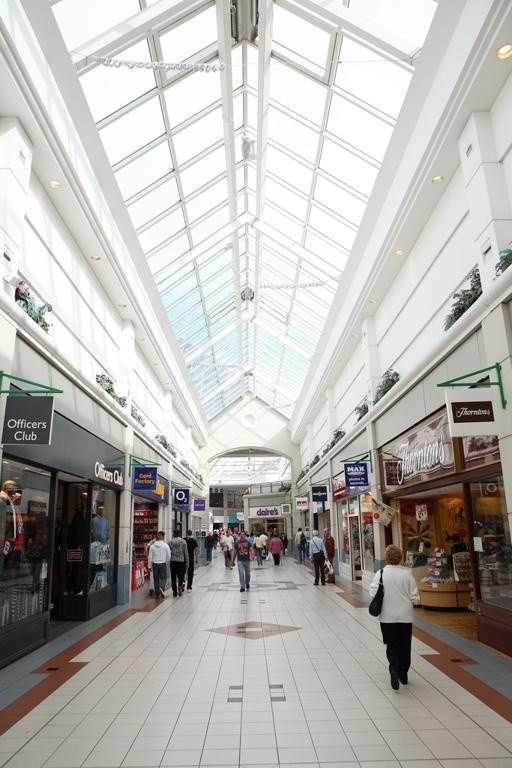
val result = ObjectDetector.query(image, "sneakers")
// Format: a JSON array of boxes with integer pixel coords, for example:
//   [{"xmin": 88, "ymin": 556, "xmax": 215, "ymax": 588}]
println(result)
[
  {"xmin": 391, "ymin": 669, "xmax": 400, "ymax": 690},
  {"xmin": 156, "ymin": 587, "xmax": 166, "ymax": 599},
  {"xmin": 246, "ymin": 585, "xmax": 249, "ymax": 589},
  {"xmin": 240, "ymin": 588, "xmax": 245, "ymax": 592},
  {"xmin": 173, "ymin": 584, "xmax": 192, "ymax": 596}
]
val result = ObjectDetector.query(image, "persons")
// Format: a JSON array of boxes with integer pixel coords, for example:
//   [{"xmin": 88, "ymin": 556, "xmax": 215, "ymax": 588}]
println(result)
[
  {"xmin": 144, "ymin": 526, "xmax": 375, "ymax": 600},
  {"xmin": 209, "ymin": 488, "xmax": 223, "ymax": 507},
  {"xmin": 1, "ymin": 480, "xmax": 110, "ymax": 624},
  {"xmin": 370, "ymin": 543, "xmax": 422, "ymax": 691}
]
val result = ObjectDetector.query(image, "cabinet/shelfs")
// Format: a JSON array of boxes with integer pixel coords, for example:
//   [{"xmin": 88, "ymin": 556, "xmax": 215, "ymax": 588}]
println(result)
[
  {"xmin": 416, "ymin": 539, "xmax": 475, "ymax": 612},
  {"xmin": 133, "ymin": 510, "xmax": 158, "ymax": 561}
]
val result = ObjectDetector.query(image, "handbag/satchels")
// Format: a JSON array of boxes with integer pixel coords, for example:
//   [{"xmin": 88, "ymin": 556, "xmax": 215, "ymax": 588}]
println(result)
[
  {"xmin": 313, "ymin": 551, "xmax": 325, "ymax": 564},
  {"xmin": 301, "ymin": 534, "xmax": 306, "ymax": 545},
  {"xmin": 323, "ymin": 560, "xmax": 335, "ymax": 576},
  {"xmin": 370, "ymin": 584, "xmax": 384, "ymax": 616}
]
